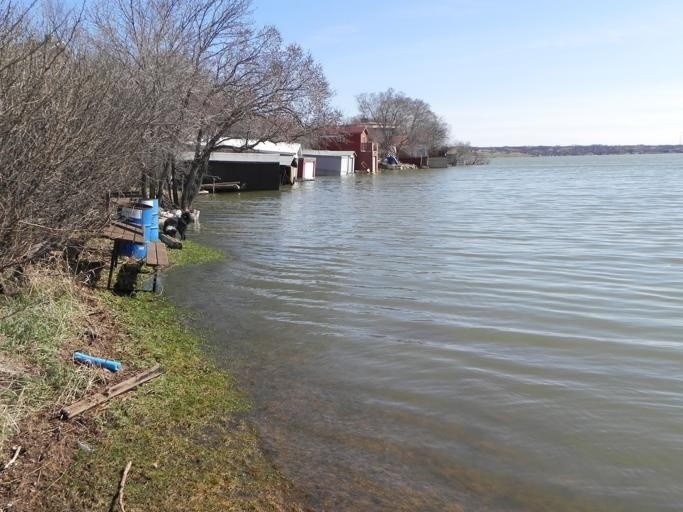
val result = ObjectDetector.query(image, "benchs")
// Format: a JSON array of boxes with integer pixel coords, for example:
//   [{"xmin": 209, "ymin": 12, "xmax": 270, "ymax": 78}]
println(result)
[{"xmin": 146, "ymin": 242, "xmax": 168, "ymax": 293}]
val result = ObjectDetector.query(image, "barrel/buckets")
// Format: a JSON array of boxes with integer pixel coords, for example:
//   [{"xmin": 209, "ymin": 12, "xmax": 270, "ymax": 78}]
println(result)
[
  {"xmin": 116, "ymin": 202, "xmax": 153, "ymax": 258},
  {"xmin": 138, "ymin": 199, "xmax": 159, "ymax": 241}
]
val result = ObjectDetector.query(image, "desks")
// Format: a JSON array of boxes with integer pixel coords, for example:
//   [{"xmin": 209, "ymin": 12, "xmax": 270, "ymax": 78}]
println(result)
[{"xmin": 95, "ymin": 221, "xmax": 145, "ymax": 288}]
